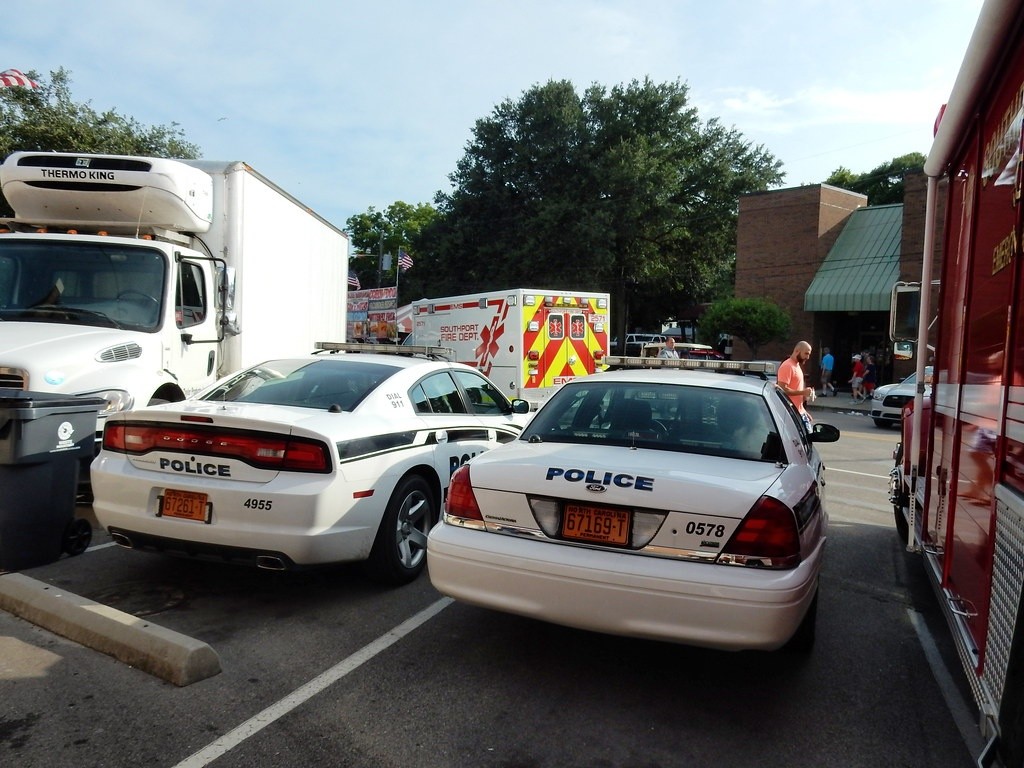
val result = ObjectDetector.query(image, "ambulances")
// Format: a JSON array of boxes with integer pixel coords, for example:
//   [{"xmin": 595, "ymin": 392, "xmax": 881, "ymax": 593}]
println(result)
[{"xmin": 399, "ymin": 289, "xmax": 612, "ymax": 415}]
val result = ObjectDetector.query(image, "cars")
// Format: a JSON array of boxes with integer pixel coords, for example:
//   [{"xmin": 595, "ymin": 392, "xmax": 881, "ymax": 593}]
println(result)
[
  {"xmin": 613, "ymin": 333, "xmax": 724, "ymax": 360},
  {"xmin": 427, "ymin": 354, "xmax": 841, "ymax": 657},
  {"xmin": 89, "ymin": 340, "xmax": 532, "ymax": 591},
  {"xmin": 870, "ymin": 365, "xmax": 935, "ymax": 428}
]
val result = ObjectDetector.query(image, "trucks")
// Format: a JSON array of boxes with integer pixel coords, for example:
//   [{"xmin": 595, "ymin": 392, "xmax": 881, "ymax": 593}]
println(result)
[
  {"xmin": 887, "ymin": 1, "xmax": 1024, "ymax": 768},
  {"xmin": 0, "ymin": 149, "xmax": 348, "ymax": 484}
]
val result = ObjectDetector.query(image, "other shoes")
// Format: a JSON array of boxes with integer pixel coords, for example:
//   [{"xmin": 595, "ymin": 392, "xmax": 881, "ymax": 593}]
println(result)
[
  {"xmin": 833, "ymin": 389, "xmax": 838, "ymax": 396},
  {"xmin": 861, "ymin": 397, "xmax": 866, "ymax": 404},
  {"xmin": 849, "ymin": 400, "xmax": 858, "ymax": 405},
  {"xmin": 866, "ymin": 395, "xmax": 873, "ymax": 400},
  {"xmin": 817, "ymin": 393, "xmax": 827, "ymax": 397}
]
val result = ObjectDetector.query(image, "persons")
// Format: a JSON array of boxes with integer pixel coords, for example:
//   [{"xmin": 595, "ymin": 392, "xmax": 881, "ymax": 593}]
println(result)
[
  {"xmin": 817, "ymin": 348, "xmax": 837, "ymax": 397},
  {"xmin": 777, "ymin": 341, "xmax": 813, "ymax": 434},
  {"xmin": 958, "ymin": 349, "xmax": 1004, "ymax": 506},
  {"xmin": 848, "ymin": 354, "xmax": 875, "ymax": 405},
  {"xmin": 658, "ymin": 337, "xmax": 680, "ymax": 370}
]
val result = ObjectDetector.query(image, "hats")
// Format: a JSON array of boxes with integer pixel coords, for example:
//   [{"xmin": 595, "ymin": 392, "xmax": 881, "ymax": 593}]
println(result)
[{"xmin": 852, "ymin": 355, "xmax": 862, "ymax": 361}]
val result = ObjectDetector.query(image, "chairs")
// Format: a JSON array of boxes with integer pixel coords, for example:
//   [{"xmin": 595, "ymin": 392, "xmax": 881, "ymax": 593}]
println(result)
[{"xmin": 604, "ymin": 399, "xmax": 652, "ymax": 430}]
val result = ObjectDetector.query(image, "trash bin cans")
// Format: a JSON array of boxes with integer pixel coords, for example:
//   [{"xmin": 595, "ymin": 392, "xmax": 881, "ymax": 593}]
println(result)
[{"xmin": 0, "ymin": 389, "xmax": 107, "ymax": 571}]
